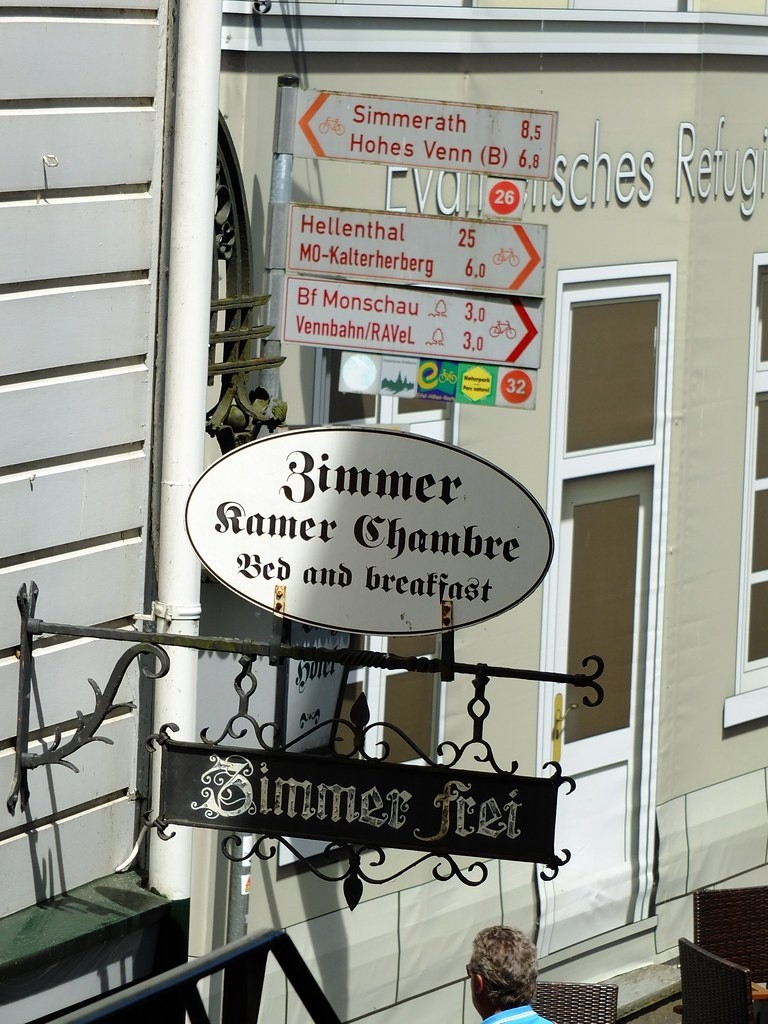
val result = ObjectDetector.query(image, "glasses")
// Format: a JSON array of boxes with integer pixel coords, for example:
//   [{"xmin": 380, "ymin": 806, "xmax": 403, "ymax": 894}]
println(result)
[{"xmin": 466, "ymin": 965, "xmax": 473, "ymax": 977}]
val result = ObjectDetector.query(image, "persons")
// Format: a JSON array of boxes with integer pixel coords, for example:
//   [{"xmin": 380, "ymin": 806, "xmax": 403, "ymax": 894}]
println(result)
[{"xmin": 465, "ymin": 925, "xmax": 559, "ymax": 1024}]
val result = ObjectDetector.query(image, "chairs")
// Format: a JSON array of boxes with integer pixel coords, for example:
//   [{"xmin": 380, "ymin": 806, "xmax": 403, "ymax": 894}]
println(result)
[{"xmin": 529, "ymin": 885, "xmax": 768, "ymax": 1024}]
